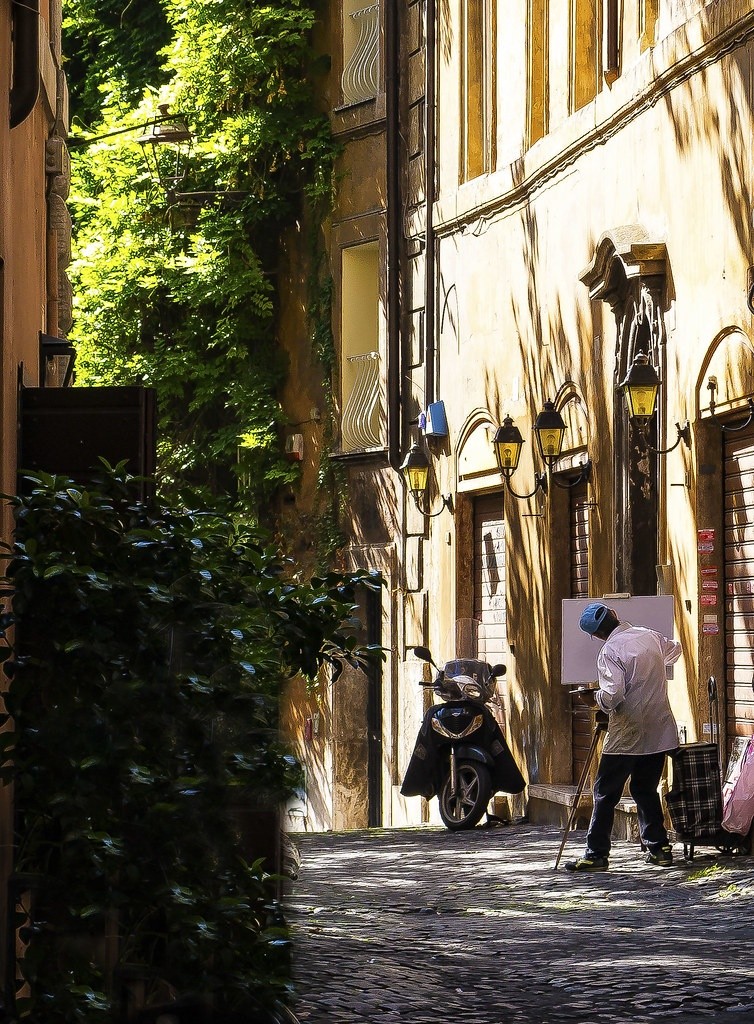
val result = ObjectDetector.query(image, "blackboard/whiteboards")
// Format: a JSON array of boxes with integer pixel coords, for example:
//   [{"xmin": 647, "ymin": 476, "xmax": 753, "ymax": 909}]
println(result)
[{"xmin": 561, "ymin": 594, "xmax": 675, "ymax": 686}]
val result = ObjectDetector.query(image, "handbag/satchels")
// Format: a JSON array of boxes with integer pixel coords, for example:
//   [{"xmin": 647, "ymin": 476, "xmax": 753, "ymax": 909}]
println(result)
[{"xmin": 721, "ymin": 738, "xmax": 754, "ymax": 837}]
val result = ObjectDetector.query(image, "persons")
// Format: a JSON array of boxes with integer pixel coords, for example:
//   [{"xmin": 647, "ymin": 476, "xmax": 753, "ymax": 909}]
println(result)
[{"xmin": 565, "ymin": 603, "xmax": 681, "ymax": 872}]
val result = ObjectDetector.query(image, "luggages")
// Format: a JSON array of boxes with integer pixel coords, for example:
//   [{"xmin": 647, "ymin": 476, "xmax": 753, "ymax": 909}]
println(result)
[{"xmin": 664, "ymin": 676, "xmax": 733, "ymax": 861}]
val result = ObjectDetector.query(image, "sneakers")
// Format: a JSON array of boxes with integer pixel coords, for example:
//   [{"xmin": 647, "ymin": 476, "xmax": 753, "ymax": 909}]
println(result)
[
  {"xmin": 645, "ymin": 845, "xmax": 672, "ymax": 866},
  {"xmin": 565, "ymin": 855, "xmax": 609, "ymax": 872}
]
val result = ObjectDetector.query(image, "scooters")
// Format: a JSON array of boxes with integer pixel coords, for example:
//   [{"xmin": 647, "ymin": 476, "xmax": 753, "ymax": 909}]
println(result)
[{"xmin": 414, "ymin": 645, "xmax": 507, "ymax": 831}]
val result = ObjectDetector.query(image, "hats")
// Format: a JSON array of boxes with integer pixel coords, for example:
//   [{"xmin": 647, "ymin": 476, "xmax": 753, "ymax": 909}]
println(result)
[{"xmin": 580, "ymin": 603, "xmax": 608, "ymax": 640}]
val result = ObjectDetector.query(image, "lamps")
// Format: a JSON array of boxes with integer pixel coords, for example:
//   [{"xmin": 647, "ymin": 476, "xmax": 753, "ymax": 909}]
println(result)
[
  {"xmin": 620, "ymin": 350, "xmax": 692, "ymax": 454},
  {"xmin": 531, "ymin": 398, "xmax": 593, "ymax": 490},
  {"xmin": 397, "ymin": 441, "xmax": 453, "ymax": 517},
  {"xmin": 492, "ymin": 414, "xmax": 548, "ymax": 500},
  {"xmin": 18, "ymin": 331, "xmax": 76, "ymax": 387},
  {"xmin": 63, "ymin": 103, "xmax": 197, "ymax": 199}
]
